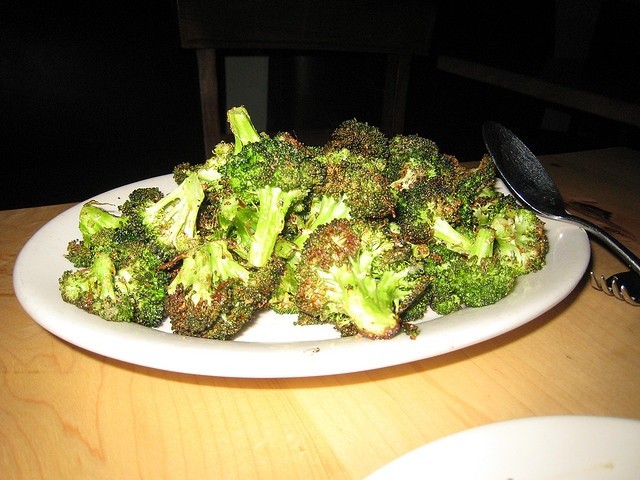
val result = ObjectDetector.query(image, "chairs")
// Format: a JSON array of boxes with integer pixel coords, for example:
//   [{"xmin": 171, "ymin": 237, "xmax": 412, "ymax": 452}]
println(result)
[{"xmin": 176, "ymin": 1, "xmax": 434, "ymax": 165}]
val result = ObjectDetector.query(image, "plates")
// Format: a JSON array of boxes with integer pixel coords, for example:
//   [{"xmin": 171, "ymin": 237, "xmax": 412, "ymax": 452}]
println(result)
[
  {"xmin": 359, "ymin": 415, "xmax": 639, "ymax": 476},
  {"xmin": 11, "ymin": 165, "xmax": 591, "ymax": 378}
]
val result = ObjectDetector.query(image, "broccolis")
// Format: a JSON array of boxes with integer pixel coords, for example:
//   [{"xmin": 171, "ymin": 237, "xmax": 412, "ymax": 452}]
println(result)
[{"xmin": 57, "ymin": 105, "xmax": 547, "ymax": 344}]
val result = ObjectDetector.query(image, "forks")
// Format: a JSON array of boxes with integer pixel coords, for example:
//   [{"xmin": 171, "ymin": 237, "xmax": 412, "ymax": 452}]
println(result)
[{"xmin": 589, "ymin": 263, "xmax": 640, "ymax": 308}]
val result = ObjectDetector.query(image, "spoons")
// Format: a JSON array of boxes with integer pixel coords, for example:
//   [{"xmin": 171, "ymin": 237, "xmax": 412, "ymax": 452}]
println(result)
[{"xmin": 481, "ymin": 121, "xmax": 639, "ymax": 282}]
